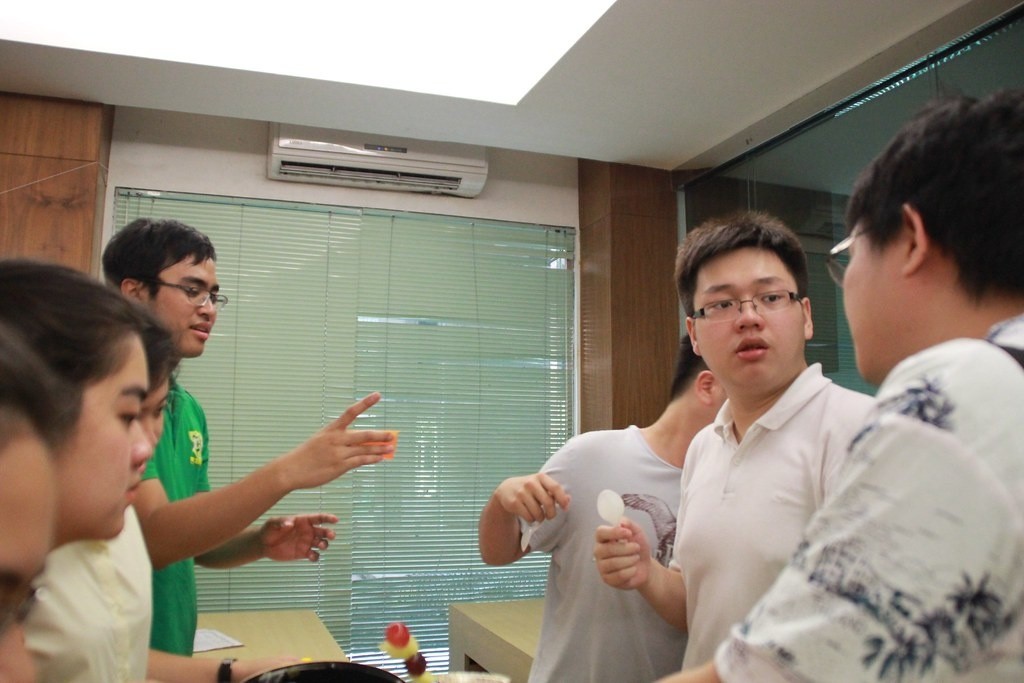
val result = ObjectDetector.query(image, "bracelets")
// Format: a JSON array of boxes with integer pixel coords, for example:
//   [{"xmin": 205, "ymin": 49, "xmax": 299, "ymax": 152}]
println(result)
[{"xmin": 217, "ymin": 658, "xmax": 237, "ymax": 683}]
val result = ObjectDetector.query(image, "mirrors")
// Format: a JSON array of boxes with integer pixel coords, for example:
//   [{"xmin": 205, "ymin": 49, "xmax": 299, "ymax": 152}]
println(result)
[{"xmin": 683, "ymin": 0, "xmax": 1023, "ymax": 396}]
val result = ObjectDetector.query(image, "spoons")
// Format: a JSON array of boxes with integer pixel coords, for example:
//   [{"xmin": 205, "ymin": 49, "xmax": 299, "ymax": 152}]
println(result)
[{"xmin": 597, "ymin": 490, "xmax": 628, "ymax": 543}]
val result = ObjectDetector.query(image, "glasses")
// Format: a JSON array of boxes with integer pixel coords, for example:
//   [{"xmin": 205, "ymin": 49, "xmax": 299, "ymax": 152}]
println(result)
[
  {"xmin": 692, "ymin": 289, "xmax": 802, "ymax": 324},
  {"xmin": 134, "ymin": 274, "xmax": 228, "ymax": 311},
  {"xmin": 0, "ymin": 586, "xmax": 43, "ymax": 633},
  {"xmin": 825, "ymin": 211, "xmax": 903, "ymax": 288}
]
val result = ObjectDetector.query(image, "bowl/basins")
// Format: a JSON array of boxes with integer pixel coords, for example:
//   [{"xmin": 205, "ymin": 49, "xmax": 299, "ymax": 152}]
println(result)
[
  {"xmin": 348, "ymin": 430, "xmax": 400, "ymax": 459},
  {"xmin": 244, "ymin": 661, "xmax": 405, "ymax": 683}
]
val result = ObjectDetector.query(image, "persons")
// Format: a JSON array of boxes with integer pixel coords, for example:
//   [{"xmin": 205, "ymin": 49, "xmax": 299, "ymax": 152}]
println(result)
[
  {"xmin": 0, "ymin": 218, "xmax": 395, "ymax": 683},
  {"xmin": 478, "ymin": 88, "xmax": 1024, "ymax": 683}
]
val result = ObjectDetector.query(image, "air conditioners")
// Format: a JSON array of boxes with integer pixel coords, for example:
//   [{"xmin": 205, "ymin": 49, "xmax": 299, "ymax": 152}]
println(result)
[
  {"xmin": 267, "ymin": 121, "xmax": 488, "ymax": 198},
  {"xmin": 789, "ymin": 192, "xmax": 851, "ymax": 241}
]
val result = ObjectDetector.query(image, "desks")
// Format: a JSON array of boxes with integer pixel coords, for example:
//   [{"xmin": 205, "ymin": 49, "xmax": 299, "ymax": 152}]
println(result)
[
  {"xmin": 191, "ymin": 610, "xmax": 351, "ymax": 662},
  {"xmin": 448, "ymin": 598, "xmax": 543, "ymax": 683}
]
